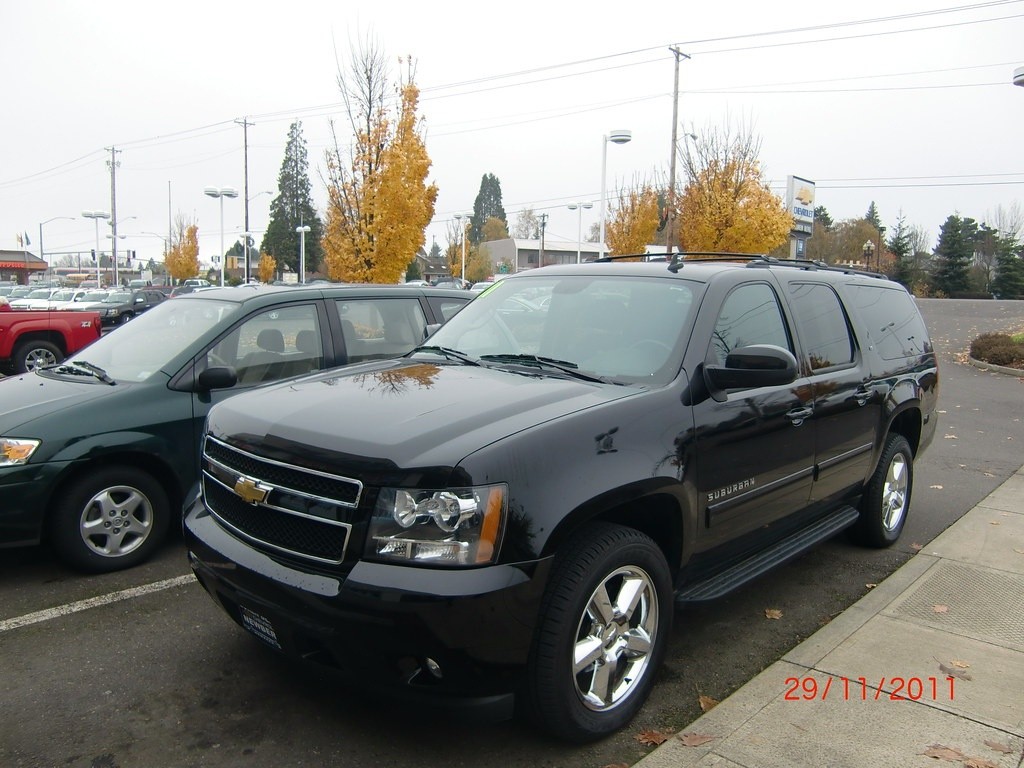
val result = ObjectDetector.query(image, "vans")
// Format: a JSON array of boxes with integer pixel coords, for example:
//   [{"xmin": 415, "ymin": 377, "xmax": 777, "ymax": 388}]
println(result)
[
  {"xmin": 128, "ymin": 279, "xmax": 152, "ymax": 289},
  {"xmin": 0, "ymin": 281, "xmax": 519, "ymax": 570},
  {"xmin": 184, "ymin": 278, "xmax": 211, "ymax": 289}
]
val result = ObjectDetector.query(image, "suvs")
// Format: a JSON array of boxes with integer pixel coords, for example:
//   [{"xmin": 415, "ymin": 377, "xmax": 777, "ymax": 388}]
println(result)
[
  {"xmin": 85, "ymin": 291, "xmax": 173, "ymax": 329},
  {"xmin": 5, "ymin": 289, "xmax": 118, "ymax": 313},
  {"xmin": 178, "ymin": 251, "xmax": 941, "ymax": 744}
]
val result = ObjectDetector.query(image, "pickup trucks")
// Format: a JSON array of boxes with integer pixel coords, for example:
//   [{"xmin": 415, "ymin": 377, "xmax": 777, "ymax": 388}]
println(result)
[{"xmin": 0, "ymin": 296, "xmax": 103, "ymax": 377}]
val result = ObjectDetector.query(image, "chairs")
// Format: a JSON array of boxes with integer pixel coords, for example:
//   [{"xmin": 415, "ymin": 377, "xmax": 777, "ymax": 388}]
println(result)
[
  {"xmin": 340, "ymin": 319, "xmax": 365, "ymax": 361},
  {"xmin": 234, "ymin": 328, "xmax": 285, "ymax": 382},
  {"xmin": 570, "ymin": 297, "xmax": 627, "ymax": 360},
  {"xmin": 279, "ymin": 329, "xmax": 317, "ymax": 378},
  {"xmin": 379, "ymin": 320, "xmax": 417, "ymax": 357}
]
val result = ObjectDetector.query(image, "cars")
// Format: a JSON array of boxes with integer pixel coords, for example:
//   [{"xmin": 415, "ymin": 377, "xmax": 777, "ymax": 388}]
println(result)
[
  {"xmin": 406, "ymin": 277, "xmax": 473, "ymax": 290},
  {"xmin": 0, "ymin": 286, "xmax": 15, "ymax": 297},
  {"xmin": 170, "ymin": 286, "xmax": 193, "ymax": 298}
]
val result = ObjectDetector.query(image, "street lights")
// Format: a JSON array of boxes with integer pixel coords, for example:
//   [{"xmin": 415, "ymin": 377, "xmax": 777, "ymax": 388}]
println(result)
[
  {"xmin": 453, "ymin": 211, "xmax": 475, "ymax": 286},
  {"xmin": 295, "ymin": 225, "xmax": 311, "ymax": 285},
  {"xmin": 106, "ymin": 233, "xmax": 127, "ymax": 285},
  {"xmin": 567, "ymin": 200, "xmax": 594, "ymax": 264},
  {"xmin": 597, "ymin": 127, "xmax": 632, "ymax": 259},
  {"xmin": 203, "ymin": 186, "xmax": 240, "ymax": 289},
  {"xmin": 240, "ymin": 231, "xmax": 253, "ymax": 284},
  {"xmin": 39, "ymin": 217, "xmax": 75, "ymax": 261},
  {"xmin": 81, "ymin": 210, "xmax": 111, "ymax": 289}
]
